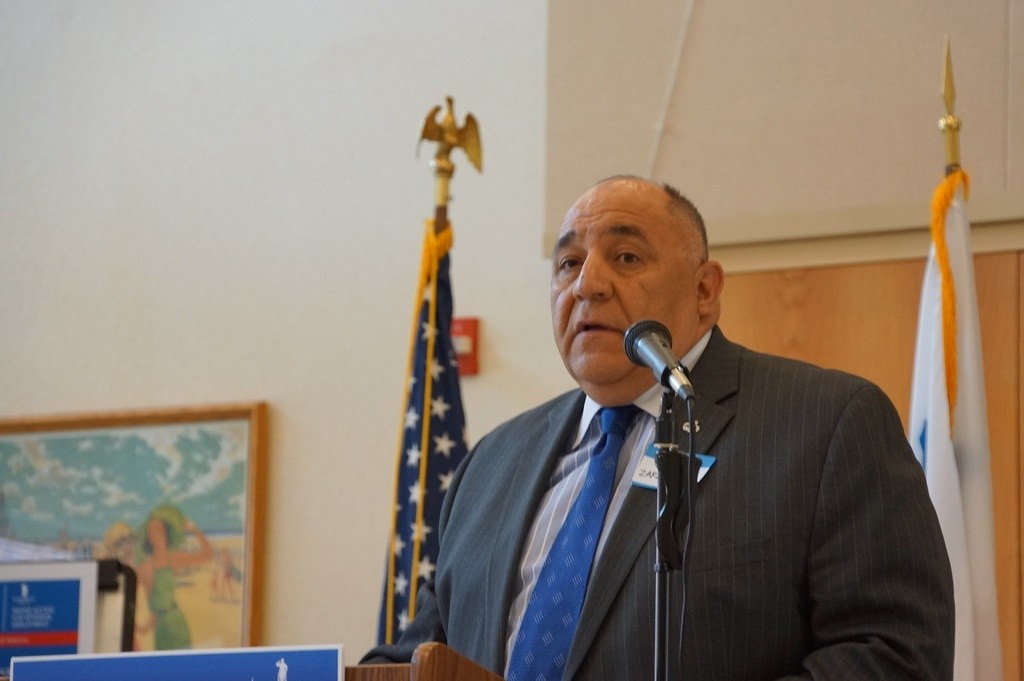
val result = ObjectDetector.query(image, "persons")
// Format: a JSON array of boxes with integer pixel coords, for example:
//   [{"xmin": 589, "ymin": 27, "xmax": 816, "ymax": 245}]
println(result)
[{"xmin": 358, "ymin": 173, "xmax": 956, "ymax": 681}]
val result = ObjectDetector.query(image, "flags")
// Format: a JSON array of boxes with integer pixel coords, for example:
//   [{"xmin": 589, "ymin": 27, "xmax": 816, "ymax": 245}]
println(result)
[
  {"xmin": 369, "ymin": 214, "xmax": 469, "ymax": 655},
  {"xmin": 906, "ymin": 172, "xmax": 1005, "ymax": 681}
]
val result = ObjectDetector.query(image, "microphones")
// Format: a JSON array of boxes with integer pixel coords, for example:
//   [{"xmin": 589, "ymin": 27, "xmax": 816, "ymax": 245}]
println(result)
[{"xmin": 623, "ymin": 320, "xmax": 694, "ymax": 401}]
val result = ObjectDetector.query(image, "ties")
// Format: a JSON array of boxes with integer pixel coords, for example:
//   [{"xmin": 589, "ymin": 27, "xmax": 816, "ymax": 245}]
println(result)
[{"xmin": 504, "ymin": 403, "xmax": 641, "ymax": 680}]
